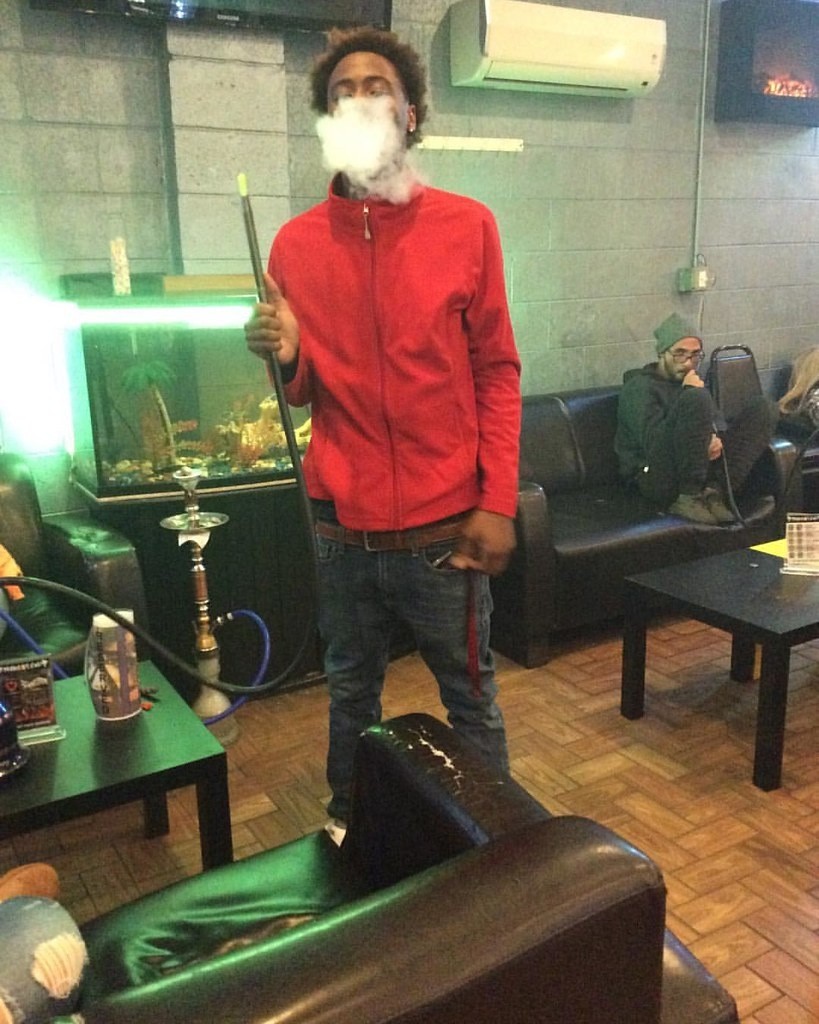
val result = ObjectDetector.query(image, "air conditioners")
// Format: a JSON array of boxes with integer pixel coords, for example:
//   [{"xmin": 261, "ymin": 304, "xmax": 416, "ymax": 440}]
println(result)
[{"xmin": 449, "ymin": 0, "xmax": 669, "ymax": 100}]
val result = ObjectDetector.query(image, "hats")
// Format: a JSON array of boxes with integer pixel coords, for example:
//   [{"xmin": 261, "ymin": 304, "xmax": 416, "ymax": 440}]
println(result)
[{"xmin": 652, "ymin": 311, "xmax": 703, "ymax": 354}]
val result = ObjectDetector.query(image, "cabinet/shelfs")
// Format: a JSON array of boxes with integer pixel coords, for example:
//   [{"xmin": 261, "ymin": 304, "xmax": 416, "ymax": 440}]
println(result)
[{"xmin": 68, "ymin": 478, "xmax": 418, "ymax": 704}]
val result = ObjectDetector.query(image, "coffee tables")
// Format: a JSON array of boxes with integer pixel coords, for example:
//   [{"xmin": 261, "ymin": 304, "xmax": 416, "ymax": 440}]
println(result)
[
  {"xmin": 621, "ymin": 549, "xmax": 819, "ymax": 790},
  {"xmin": 0, "ymin": 661, "xmax": 234, "ymax": 872}
]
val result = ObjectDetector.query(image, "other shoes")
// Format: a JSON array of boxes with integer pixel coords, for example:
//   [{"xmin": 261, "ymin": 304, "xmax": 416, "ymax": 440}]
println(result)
[
  {"xmin": 668, "ymin": 492, "xmax": 720, "ymax": 525},
  {"xmin": 324, "ymin": 816, "xmax": 349, "ymax": 848},
  {"xmin": 702, "ymin": 489, "xmax": 736, "ymax": 523}
]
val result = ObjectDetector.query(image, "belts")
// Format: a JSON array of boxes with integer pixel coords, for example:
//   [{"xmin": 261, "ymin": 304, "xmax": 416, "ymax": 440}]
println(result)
[{"xmin": 313, "ymin": 516, "xmax": 464, "ymax": 552}]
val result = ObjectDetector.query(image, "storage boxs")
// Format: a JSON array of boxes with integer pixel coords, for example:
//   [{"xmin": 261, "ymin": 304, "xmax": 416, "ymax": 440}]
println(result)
[{"xmin": 59, "ymin": 295, "xmax": 312, "ymax": 503}]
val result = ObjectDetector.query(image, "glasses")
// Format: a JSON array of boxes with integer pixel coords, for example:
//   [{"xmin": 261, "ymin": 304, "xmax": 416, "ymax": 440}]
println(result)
[{"xmin": 666, "ymin": 347, "xmax": 706, "ymax": 363}]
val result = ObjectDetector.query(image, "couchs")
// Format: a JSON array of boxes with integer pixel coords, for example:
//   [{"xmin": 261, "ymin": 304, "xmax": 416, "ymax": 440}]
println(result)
[
  {"xmin": 34, "ymin": 714, "xmax": 744, "ymax": 1024},
  {"xmin": 490, "ymin": 385, "xmax": 802, "ymax": 668},
  {"xmin": 0, "ymin": 452, "xmax": 153, "ymax": 677}
]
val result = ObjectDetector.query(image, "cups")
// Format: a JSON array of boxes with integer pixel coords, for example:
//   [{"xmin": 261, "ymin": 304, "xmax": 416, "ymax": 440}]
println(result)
[{"xmin": 84, "ymin": 609, "xmax": 142, "ymax": 721}]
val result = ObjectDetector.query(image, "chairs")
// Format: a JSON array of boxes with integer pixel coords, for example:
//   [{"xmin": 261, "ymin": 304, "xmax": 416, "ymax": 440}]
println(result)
[{"xmin": 705, "ymin": 343, "xmax": 819, "ymax": 475}]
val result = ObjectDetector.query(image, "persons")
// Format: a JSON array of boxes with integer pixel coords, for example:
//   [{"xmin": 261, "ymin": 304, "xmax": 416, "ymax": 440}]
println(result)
[
  {"xmin": 779, "ymin": 347, "xmax": 819, "ymax": 426},
  {"xmin": 0, "ymin": 862, "xmax": 91, "ymax": 1024},
  {"xmin": 244, "ymin": 27, "xmax": 521, "ymax": 847},
  {"xmin": 613, "ymin": 313, "xmax": 735, "ymax": 525}
]
